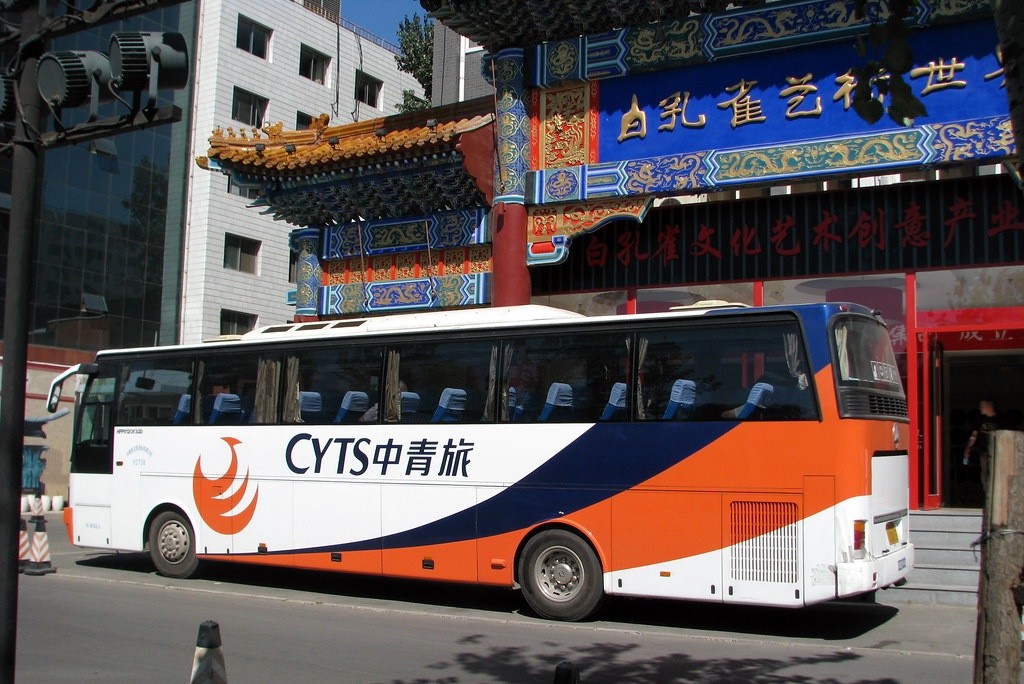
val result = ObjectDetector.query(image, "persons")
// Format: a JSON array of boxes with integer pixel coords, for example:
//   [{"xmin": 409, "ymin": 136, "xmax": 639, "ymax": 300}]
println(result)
[
  {"xmin": 963, "ymin": 401, "xmax": 998, "ymax": 494},
  {"xmin": 720, "ymin": 374, "xmax": 776, "ymax": 417},
  {"xmin": 358, "ymin": 379, "xmax": 408, "ymax": 423}
]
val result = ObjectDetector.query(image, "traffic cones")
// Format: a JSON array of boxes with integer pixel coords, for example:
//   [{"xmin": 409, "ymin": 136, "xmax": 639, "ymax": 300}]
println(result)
[
  {"xmin": 23, "ymin": 515, "xmax": 57, "ymax": 577},
  {"xmin": 190, "ymin": 621, "xmax": 226, "ymax": 684},
  {"xmin": 17, "ymin": 518, "xmax": 31, "ymax": 574}
]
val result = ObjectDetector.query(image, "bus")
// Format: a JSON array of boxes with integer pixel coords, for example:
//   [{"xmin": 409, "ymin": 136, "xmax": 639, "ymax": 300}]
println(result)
[{"xmin": 45, "ymin": 302, "xmax": 915, "ymax": 625}]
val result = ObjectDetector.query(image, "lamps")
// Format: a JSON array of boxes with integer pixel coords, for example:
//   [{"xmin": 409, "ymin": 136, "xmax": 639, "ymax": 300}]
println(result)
[
  {"xmin": 34, "ymin": 50, "xmax": 120, "ymax": 132},
  {"xmin": 426, "ymin": 119, "xmax": 438, "ymax": 133},
  {"xmin": 286, "ymin": 144, "xmax": 297, "ymax": 156},
  {"xmin": 108, "ymin": 29, "xmax": 190, "ymax": 114},
  {"xmin": 0, "ymin": 72, "xmax": 16, "ymax": 133},
  {"xmin": 375, "ymin": 128, "xmax": 387, "ymax": 142},
  {"xmin": 328, "ymin": 136, "xmax": 339, "ymax": 150},
  {"xmin": 255, "ymin": 143, "xmax": 266, "ymax": 159}
]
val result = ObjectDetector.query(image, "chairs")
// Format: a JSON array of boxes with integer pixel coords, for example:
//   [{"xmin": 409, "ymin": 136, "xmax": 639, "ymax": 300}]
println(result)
[
  {"xmin": 321, "ymin": 391, "xmax": 343, "ymax": 424},
  {"xmin": 206, "ymin": 393, "xmax": 242, "ymax": 425},
  {"xmin": 332, "ymin": 391, "xmax": 370, "ymax": 424},
  {"xmin": 662, "ymin": 378, "xmax": 696, "ymax": 419},
  {"xmin": 299, "ymin": 391, "xmax": 322, "ymax": 424},
  {"xmin": 599, "ymin": 381, "xmax": 628, "ymax": 419},
  {"xmin": 431, "ymin": 387, "xmax": 468, "ymax": 422},
  {"xmin": 736, "ymin": 382, "xmax": 775, "ymax": 419},
  {"xmin": 238, "ymin": 392, "xmax": 255, "ymax": 425},
  {"xmin": 172, "ymin": 394, "xmax": 191, "ymax": 426},
  {"xmin": 509, "ymin": 386, "xmax": 517, "ymax": 420},
  {"xmin": 562, "ymin": 383, "xmax": 591, "ymax": 421},
  {"xmin": 512, "ymin": 390, "xmax": 530, "ymax": 421},
  {"xmin": 401, "ymin": 391, "xmax": 421, "ymax": 422},
  {"xmin": 536, "ymin": 382, "xmax": 573, "ymax": 420}
]
